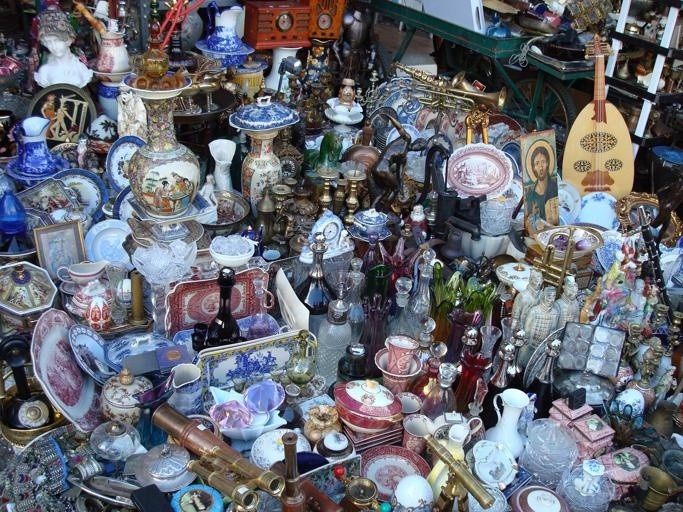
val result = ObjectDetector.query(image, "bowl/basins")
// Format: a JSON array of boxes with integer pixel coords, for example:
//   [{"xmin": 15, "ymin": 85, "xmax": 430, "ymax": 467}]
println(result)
[{"xmin": 533, "ymin": 225, "xmax": 603, "ymax": 262}]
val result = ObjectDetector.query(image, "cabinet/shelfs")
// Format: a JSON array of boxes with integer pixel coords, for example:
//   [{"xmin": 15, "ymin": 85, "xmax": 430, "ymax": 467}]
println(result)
[{"xmin": 604, "ymin": 0, "xmax": 683, "ymax": 192}]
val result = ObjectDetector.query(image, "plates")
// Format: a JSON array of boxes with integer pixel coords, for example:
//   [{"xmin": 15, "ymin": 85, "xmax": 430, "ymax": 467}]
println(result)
[
  {"xmin": 29, "ymin": 307, "xmax": 179, "ymax": 433},
  {"xmin": 375, "ymin": 87, "xmax": 620, "ymax": 232},
  {"xmin": 342, "ymin": 423, "xmax": 434, "ymax": 510},
  {"xmin": 51, "ymin": 134, "xmax": 214, "ymax": 273},
  {"xmin": 124, "ymin": 71, "xmax": 192, "ymax": 100}
]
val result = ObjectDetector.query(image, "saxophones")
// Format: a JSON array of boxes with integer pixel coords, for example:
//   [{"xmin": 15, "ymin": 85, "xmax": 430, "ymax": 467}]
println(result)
[{"xmin": 394, "ymin": 61, "xmax": 482, "ymax": 93}]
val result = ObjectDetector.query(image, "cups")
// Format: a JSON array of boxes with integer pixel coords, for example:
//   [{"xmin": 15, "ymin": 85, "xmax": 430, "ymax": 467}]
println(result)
[
  {"xmin": 633, "ymin": 449, "xmax": 683, "ymax": 510},
  {"xmin": 481, "ymin": 201, "xmax": 513, "ymax": 237},
  {"xmin": 371, "ymin": 335, "xmax": 433, "ymax": 455}
]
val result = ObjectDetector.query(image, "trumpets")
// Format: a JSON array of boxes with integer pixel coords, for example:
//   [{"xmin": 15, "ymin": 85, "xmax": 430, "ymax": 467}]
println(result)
[{"xmin": 385, "ymin": 78, "xmax": 506, "ymax": 113}]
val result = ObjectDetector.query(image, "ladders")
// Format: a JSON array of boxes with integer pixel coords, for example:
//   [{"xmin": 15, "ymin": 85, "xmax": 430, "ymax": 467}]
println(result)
[{"xmin": 604, "ymin": 0, "xmax": 680, "ymax": 162}]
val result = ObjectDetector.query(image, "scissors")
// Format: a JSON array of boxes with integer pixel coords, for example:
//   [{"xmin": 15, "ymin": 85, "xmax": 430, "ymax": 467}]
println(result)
[{"xmin": 609, "ymin": 399, "xmax": 632, "ymax": 419}]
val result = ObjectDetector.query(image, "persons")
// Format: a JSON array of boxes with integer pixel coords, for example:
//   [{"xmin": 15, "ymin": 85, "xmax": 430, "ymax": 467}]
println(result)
[
  {"xmin": 512, "ymin": 268, "xmax": 579, "ymax": 368},
  {"xmin": 34, "ymin": 11, "xmax": 93, "ymax": 89},
  {"xmin": 525, "ymin": 147, "xmax": 559, "ymax": 236},
  {"xmin": 41, "ymin": 94, "xmax": 88, "ymax": 167},
  {"xmin": 117, "ymin": 154, "xmax": 194, "ymax": 213},
  {"xmin": 189, "ymin": 491, "xmax": 207, "ymax": 511},
  {"xmin": 51, "ymin": 241, "xmax": 70, "ymax": 272},
  {"xmin": 77, "ymin": 343, "xmax": 94, "ymax": 367},
  {"xmin": 29, "ymin": 193, "xmax": 68, "ymax": 213}
]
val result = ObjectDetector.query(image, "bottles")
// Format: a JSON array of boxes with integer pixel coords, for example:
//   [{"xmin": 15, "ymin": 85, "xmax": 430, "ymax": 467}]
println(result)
[{"xmin": 446, "ymin": 388, "xmax": 530, "ymax": 460}]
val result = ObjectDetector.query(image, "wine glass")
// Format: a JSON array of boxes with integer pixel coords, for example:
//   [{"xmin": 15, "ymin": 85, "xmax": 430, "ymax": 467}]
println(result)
[{"xmin": 197, "ymin": 69, "xmax": 222, "ymax": 114}]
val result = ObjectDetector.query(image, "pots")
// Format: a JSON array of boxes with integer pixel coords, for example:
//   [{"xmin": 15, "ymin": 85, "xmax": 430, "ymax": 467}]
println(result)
[{"xmin": 333, "ymin": 378, "xmax": 403, "ymax": 435}]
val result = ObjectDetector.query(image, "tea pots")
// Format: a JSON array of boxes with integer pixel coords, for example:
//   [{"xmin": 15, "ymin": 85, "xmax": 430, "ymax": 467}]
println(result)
[
  {"xmin": 11, "ymin": 117, "xmax": 55, "ymax": 178},
  {"xmin": 95, "ymin": 32, "xmax": 132, "ymax": 73}
]
